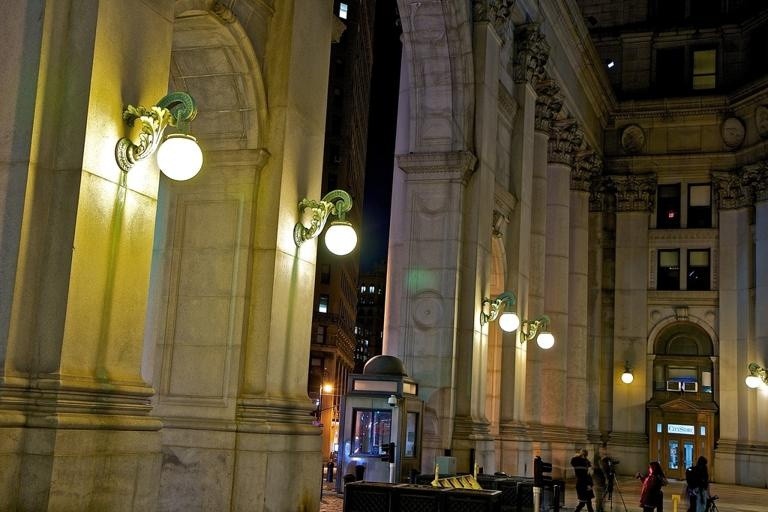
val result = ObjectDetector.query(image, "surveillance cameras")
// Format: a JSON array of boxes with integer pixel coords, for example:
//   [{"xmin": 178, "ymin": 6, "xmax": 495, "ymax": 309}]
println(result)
[{"xmin": 388, "ymin": 398, "xmax": 397, "ymax": 407}]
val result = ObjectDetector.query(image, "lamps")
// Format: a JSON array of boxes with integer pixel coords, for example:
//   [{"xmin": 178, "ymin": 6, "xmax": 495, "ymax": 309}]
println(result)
[
  {"xmin": 621, "ymin": 360, "xmax": 635, "ymax": 385},
  {"xmin": 478, "ymin": 291, "xmax": 555, "ymax": 352},
  {"xmin": 112, "ymin": 89, "xmax": 203, "ymax": 183},
  {"xmin": 292, "ymin": 190, "xmax": 359, "ymax": 256}
]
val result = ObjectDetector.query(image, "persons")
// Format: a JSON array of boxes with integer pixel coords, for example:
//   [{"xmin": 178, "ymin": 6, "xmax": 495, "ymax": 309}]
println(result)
[
  {"xmin": 638, "ymin": 462, "xmax": 669, "ymax": 512},
  {"xmin": 570, "ymin": 449, "xmax": 595, "ymax": 512},
  {"xmin": 685, "ymin": 455, "xmax": 709, "ymax": 512},
  {"xmin": 593, "ymin": 441, "xmax": 610, "ymax": 512}
]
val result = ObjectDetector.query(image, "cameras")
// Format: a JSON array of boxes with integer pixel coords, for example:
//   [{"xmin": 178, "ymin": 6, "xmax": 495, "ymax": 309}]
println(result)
[{"xmin": 608, "ymin": 460, "xmax": 619, "ymax": 467}]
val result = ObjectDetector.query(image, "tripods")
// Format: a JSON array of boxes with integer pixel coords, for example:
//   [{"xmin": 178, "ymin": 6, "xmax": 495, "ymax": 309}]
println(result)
[{"xmin": 600, "ymin": 468, "xmax": 628, "ymax": 512}]
